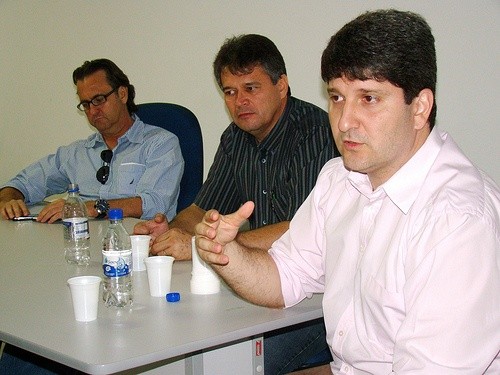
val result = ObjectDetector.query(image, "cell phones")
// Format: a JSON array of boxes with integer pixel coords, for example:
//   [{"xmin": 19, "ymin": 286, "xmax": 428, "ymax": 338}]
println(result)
[{"xmin": 13, "ymin": 214, "xmax": 40, "ymax": 221}]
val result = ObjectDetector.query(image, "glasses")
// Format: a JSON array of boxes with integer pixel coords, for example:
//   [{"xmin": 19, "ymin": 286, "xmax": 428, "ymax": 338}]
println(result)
[
  {"xmin": 96, "ymin": 150, "xmax": 113, "ymax": 184},
  {"xmin": 77, "ymin": 85, "xmax": 120, "ymax": 111}
]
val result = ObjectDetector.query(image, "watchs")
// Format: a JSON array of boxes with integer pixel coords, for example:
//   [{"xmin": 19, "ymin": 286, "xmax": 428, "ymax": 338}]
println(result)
[{"xmin": 94, "ymin": 199, "xmax": 111, "ymax": 220}]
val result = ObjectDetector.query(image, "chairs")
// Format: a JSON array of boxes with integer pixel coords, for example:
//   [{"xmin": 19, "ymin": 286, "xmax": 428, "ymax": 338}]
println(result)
[{"xmin": 132, "ymin": 103, "xmax": 203, "ymax": 213}]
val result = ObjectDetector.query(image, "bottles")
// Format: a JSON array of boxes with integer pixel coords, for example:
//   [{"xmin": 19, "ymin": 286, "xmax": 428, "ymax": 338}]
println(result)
[
  {"xmin": 101, "ymin": 208, "xmax": 135, "ymax": 309},
  {"xmin": 63, "ymin": 184, "xmax": 92, "ymax": 266}
]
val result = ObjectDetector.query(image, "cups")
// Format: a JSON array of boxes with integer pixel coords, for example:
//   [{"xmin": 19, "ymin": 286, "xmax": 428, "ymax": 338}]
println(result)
[
  {"xmin": 144, "ymin": 255, "xmax": 175, "ymax": 296},
  {"xmin": 67, "ymin": 276, "xmax": 101, "ymax": 323},
  {"xmin": 129, "ymin": 235, "xmax": 152, "ymax": 271},
  {"xmin": 189, "ymin": 236, "xmax": 219, "ymax": 294}
]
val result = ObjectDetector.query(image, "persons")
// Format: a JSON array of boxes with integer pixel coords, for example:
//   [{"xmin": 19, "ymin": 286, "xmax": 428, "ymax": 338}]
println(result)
[
  {"xmin": 128, "ymin": 34, "xmax": 342, "ymax": 375},
  {"xmin": 0, "ymin": 58, "xmax": 185, "ymax": 375},
  {"xmin": 194, "ymin": 9, "xmax": 500, "ymax": 375}
]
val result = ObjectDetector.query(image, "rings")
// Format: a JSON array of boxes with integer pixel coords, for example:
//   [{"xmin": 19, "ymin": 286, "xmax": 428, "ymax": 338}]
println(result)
[{"xmin": 163, "ymin": 250, "xmax": 167, "ymax": 256}]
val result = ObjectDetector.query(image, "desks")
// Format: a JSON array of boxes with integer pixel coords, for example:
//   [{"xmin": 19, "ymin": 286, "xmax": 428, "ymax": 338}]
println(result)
[{"xmin": 1, "ymin": 213, "xmax": 324, "ymax": 375}]
